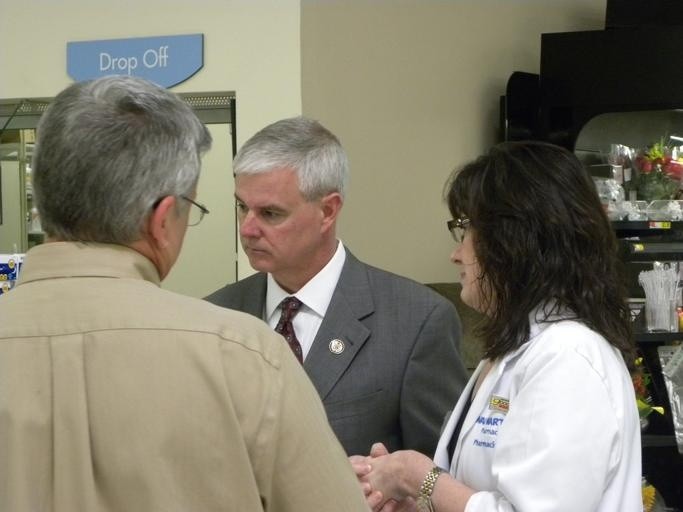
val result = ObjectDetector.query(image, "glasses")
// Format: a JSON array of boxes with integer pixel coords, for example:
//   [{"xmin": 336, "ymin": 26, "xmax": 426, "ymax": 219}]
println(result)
[
  {"xmin": 446, "ymin": 218, "xmax": 471, "ymax": 240},
  {"xmin": 182, "ymin": 194, "xmax": 207, "ymax": 229}
]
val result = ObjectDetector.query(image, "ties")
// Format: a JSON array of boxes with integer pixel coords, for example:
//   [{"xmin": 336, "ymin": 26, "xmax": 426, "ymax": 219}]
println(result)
[{"xmin": 273, "ymin": 296, "xmax": 303, "ymax": 364}]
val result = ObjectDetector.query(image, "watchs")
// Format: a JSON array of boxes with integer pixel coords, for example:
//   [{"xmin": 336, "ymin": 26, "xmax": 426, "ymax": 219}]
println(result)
[{"xmin": 414, "ymin": 465, "xmax": 450, "ymax": 512}]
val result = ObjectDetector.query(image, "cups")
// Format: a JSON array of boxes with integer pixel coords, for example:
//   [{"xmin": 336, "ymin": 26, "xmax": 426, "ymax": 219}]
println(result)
[{"xmin": 644, "ymin": 298, "xmax": 679, "ymax": 333}]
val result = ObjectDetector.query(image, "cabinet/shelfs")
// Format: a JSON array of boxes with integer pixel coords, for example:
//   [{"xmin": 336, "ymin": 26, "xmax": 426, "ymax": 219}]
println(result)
[{"xmin": 605, "ymin": 217, "xmax": 683, "ymax": 451}]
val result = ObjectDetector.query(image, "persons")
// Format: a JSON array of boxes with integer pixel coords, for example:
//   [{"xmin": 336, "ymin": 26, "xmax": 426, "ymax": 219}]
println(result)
[
  {"xmin": 0, "ymin": 74, "xmax": 370, "ymax": 512},
  {"xmin": 198, "ymin": 115, "xmax": 469, "ymax": 461},
  {"xmin": 348, "ymin": 141, "xmax": 644, "ymax": 512}
]
